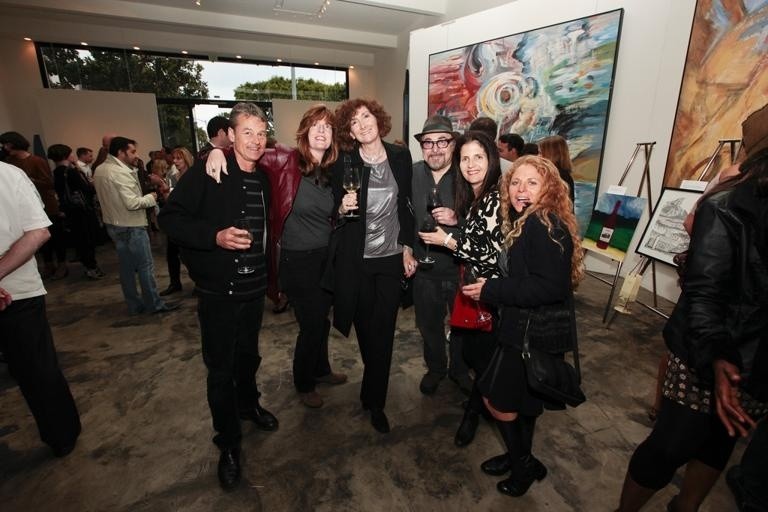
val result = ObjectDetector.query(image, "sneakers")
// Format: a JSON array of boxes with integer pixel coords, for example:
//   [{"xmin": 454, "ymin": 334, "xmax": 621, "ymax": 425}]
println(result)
[{"xmin": 420, "ymin": 371, "xmax": 447, "ymax": 395}]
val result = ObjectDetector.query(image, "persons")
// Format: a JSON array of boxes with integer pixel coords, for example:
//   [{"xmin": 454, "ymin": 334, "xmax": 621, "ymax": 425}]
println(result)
[
  {"xmin": 94, "ymin": 137, "xmax": 183, "ymax": 315},
  {"xmin": 328, "ymin": 95, "xmax": 418, "ymax": 434},
  {"xmin": 1, "ymin": 162, "xmax": 83, "ymax": 454},
  {"xmin": 156, "ymin": 104, "xmax": 273, "ymax": 493},
  {"xmin": 159, "ymin": 147, "xmax": 193, "ymax": 296},
  {"xmin": 200, "ymin": 106, "xmax": 406, "ymax": 408},
  {"xmin": 407, "ymin": 116, "xmax": 459, "ymax": 396},
  {"xmin": 419, "ymin": 117, "xmax": 586, "ymax": 495},
  {"xmin": 1, "ymin": 131, "xmax": 179, "ymax": 278},
  {"xmin": 615, "ymin": 104, "xmax": 768, "ymax": 512}
]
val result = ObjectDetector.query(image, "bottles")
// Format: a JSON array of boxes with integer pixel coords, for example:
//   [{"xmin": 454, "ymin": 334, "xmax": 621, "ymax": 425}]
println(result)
[{"xmin": 596, "ymin": 200, "xmax": 621, "ymax": 250}]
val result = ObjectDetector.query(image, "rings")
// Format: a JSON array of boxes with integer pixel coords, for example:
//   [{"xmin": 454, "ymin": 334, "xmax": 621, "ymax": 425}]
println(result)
[
  {"xmin": 212, "ymin": 169, "xmax": 216, "ymax": 173},
  {"xmin": 411, "ymin": 265, "xmax": 415, "ymax": 268}
]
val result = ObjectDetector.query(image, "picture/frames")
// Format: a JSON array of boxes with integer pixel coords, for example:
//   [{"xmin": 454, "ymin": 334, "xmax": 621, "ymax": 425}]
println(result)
[
  {"xmin": 428, "ymin": 7, "xmax": 624, "ymax": 239},
  {"xmin": 635, "ymin": 187, "xmax": 703, "ymax": 268},
  {"xmin": 662, "ymin": 0, "xmax": 768, "ymax": 189}
]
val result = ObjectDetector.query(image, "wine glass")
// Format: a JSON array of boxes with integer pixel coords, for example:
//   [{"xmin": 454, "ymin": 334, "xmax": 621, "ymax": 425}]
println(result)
[
  {"xmin": 231, "ymin": 215, "xmax": 254, "ymax": 275},
  {"xmin": 340, "ymin": 168, "xmax": 361, "ymax": 218},
  {"xmin": 452, "ymin": 261, "xmax": 494, "ymax": 324},
  {"xmin": 417, "ymin": 214, "xmax": 438, "ymax": 264}
]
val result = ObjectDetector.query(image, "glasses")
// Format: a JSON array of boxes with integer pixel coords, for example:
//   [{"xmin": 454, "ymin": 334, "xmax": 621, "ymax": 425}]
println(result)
[{"xmin": 419, "ymin": 137, "xmax": 454, "ymax": 149}]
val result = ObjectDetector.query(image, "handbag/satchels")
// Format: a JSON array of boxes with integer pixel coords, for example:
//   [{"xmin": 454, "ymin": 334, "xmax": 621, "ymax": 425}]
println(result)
[
  {"xmin": 63, "ymin": 168, "xmax": 95, "ymax": 212},
  {"xmin": 525, "ymin": 344, "xmax": 586, "ymax": 408},
  {"xmin": 450, "ymin": 289, "xmax": 493, "ymax": 332}
]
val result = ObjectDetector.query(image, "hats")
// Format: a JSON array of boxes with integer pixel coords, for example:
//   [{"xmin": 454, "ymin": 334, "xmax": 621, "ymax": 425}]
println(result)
[{"xmin": 414, "ymin": 116, "xmax": 460, "ymax": 140}]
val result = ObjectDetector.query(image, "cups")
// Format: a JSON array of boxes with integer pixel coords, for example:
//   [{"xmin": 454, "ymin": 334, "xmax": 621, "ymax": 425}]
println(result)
[
  {"xmin": 159, "ymin": 178, "xmax": 170, "ymax": 194},
  {"xmin": 425, "ymin": 186, "xmax": 443, "ymax": 214}
]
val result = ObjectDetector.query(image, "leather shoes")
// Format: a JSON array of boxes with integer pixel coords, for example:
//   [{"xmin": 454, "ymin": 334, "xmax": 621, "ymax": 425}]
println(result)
[
  {"xmin": 217, "ymin": 448, "xmax": 243, "ymax": 491},
  {"xmin": 299, "ymin": 372, "xmax": 390, "ymax": 434},
  {"xmin": 454, "ymin": 408, "xmax": 547, "ymax": 498},
  {"xmin": 152, "ymin": 303, "xmax": 181, "ymax": 314},
  {"xmin": 245, "ymin": 404, "xmax": 279, "ymax": 432},
  {"xmin": 159, "ymin": 282, "xmax": 182, "ymax": 296}
]
